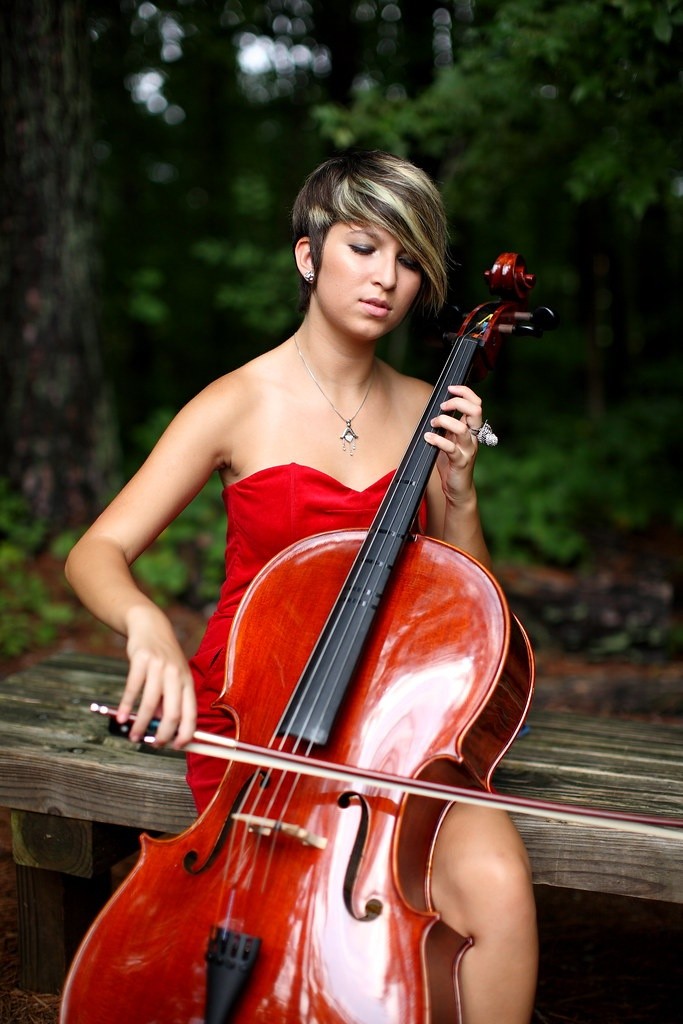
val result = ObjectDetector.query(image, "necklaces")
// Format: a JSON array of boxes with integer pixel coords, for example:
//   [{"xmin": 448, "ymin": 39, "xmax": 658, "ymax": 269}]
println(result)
[{"xmin": 293, "ymin": 328, "xmax": 376, "ymax": 456}]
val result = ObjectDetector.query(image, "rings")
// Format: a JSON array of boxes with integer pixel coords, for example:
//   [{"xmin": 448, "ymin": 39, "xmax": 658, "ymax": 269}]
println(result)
[{"xmin": 466, "ymin": 418, "xmax": 499, "ymax": 448}]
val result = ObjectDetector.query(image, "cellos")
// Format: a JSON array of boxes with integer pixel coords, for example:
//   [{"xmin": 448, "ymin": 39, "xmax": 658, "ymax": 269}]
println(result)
[{"xmin": 59, "ymin": 250, "xmax": 558, "ymax": 1024}]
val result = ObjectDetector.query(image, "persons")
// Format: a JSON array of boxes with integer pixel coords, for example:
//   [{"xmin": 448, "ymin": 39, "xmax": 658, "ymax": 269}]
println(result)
[{"xmin": 64, "ymin": 143, "xmax": 542, "ymax": 1023}]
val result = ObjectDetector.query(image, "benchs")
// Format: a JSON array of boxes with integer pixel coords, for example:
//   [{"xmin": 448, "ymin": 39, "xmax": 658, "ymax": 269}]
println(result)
[{"xmin": 1, "ymin": 650, "xmax": 683, "ymax": 994}]
